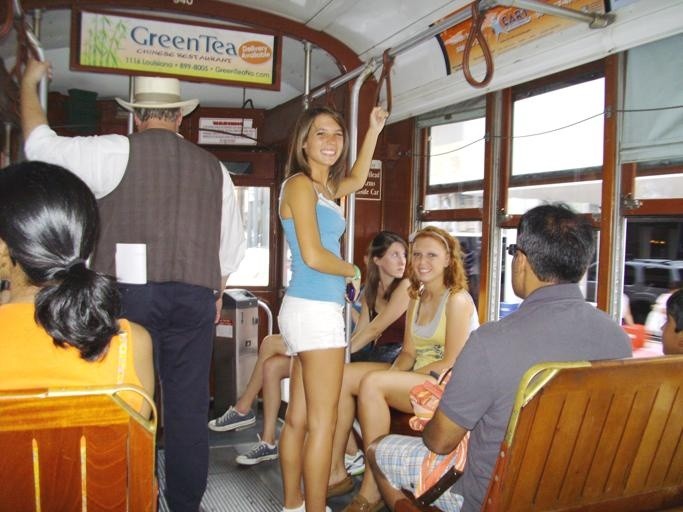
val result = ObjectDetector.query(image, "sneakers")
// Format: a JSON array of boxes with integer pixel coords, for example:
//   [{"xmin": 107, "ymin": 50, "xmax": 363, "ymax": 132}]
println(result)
[
  {"xmin": 237, "ymin": 441, "xmax": 279, "ymax": 465},
  {"xmin": 208, "ymin": 403, "xmax": 255, "ymax": 431},
  {"xmin": 344, "ymin": 449, "xmax": 365, "ymax": 474}
]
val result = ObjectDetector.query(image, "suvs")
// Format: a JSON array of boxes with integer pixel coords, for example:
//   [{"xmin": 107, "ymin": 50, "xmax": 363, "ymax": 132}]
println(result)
[{"xmin": 586, "ymin": 258, "xmax": 682, "ymax": 324}]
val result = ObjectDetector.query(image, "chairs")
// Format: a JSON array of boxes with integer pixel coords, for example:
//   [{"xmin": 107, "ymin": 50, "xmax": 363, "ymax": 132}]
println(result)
[
  {"xmin": 0, "ymin": 383, "xmax": 158, "ymax": 512},
  {"xmin": 480, "ymin": 355, "xmax": 683, "ymax": 511}
]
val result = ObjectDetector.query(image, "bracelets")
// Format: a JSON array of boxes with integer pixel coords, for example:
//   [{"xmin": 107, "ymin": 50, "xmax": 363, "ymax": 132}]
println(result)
[{"xmin": 352, "ymin": 263, "xmax": 360, "ymax": 281}]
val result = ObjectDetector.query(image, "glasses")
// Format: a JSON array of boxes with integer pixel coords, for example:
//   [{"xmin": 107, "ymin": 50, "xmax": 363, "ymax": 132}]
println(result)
[{"xmin": 508, "ymin": 243, "xmax": 526, "ymax": 256}]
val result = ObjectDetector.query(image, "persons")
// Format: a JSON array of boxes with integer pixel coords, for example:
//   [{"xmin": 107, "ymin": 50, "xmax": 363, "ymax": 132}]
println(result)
[
  {"xmin": 20, "ymin": 57, "xmax": 247, "ymax": 512},
  {"xmin": 277, "ymin": 105, "xmax": 390, "ymax": 512},
  {"xmin": 660, "ymin": 289, "xmax": 683, "ymax": 355},
  {"xmin": 0, "ymin": 162, "xmax": 155, "ymax": 422},
  {"xmin": 207, "ymin": 285, "xmax": 372, "ymax": 466},
  {"xmin": 622, "ymin": 293, "xmax": 635, "ymax": 327},
  {"xmin": 367, "ymin": 204, "xmax": 633, "ymax": 512},
  {"xmin": 344, "ymin": 232, "xmax": 413, "ymax": 476},
  {"xmin": 327, "ymin": 226, "xmax": 480, "ymax": 512}
]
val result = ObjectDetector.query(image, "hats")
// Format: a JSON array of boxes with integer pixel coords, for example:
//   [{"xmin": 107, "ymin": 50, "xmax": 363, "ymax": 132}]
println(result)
[{"xmin": 116, "ymin": 75, "xmax": 199, "ymax": 116}]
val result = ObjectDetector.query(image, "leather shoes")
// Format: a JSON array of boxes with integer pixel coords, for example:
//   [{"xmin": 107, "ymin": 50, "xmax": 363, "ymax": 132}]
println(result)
[
  {"xmin": 340, "ymin": 492, "xmax": 384, "ymax": 511},
  {"xmin": 326, "ymin": 474, "xmax": 354, "ymax": 499}
]
